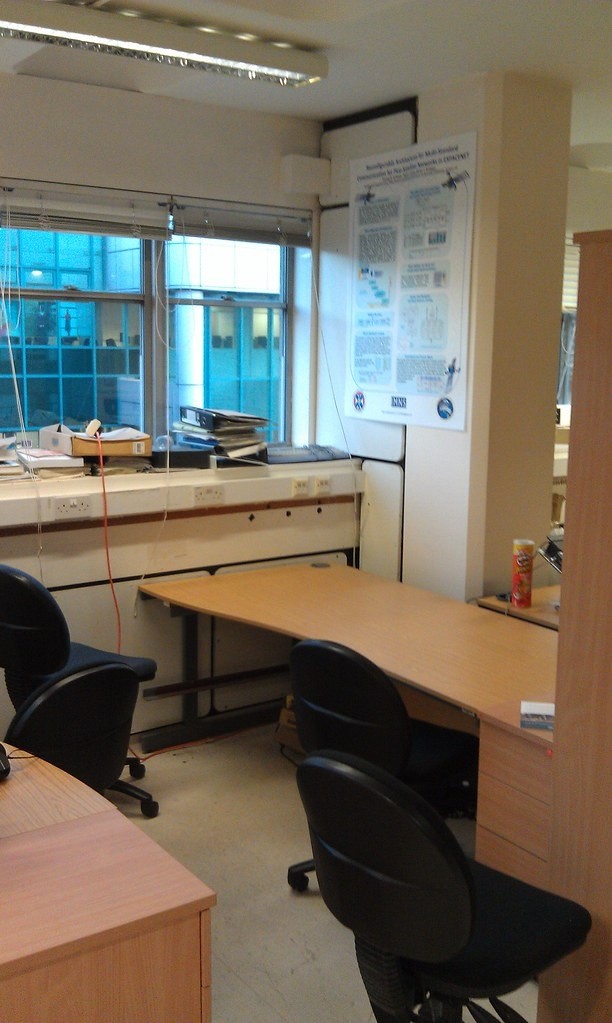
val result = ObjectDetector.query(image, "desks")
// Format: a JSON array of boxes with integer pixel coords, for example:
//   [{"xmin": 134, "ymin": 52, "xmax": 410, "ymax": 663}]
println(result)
[{"xmin": 0, "ymin": 744, "xmax": 216, "ymax": 1022}]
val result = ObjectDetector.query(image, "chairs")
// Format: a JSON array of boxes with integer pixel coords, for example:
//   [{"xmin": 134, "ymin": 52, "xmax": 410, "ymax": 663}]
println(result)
[
  {"xmin": 287, "ymin": 640, "xmax": 475, "ymax": 891},
  {"xmin": 294, "ymin": 749, "xmax": 593, "ymax": 1023},
  {"xmin": 3, "ymin": 661, "xmax": 136, "ymax": 790},
  {"xmin": 0, "ymin": 564, "xmax": 159, "ymax": 819}
]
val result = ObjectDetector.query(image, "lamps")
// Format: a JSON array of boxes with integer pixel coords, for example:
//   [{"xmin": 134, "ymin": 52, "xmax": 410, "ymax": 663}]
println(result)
[{"xmin": 1, "ymin": 1, "xmax": 329, "ymax": 89}]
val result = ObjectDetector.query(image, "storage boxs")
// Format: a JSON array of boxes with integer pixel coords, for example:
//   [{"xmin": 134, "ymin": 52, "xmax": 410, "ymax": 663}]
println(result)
[{"xmin": 39, "ymin": 424, "xmax": 152, "ymax": 457}]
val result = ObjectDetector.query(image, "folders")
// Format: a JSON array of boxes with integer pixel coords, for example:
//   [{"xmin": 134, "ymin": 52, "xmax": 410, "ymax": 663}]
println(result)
[
  {"xmin": 179, "ymin": 437, "xmax": 264, "ymax": 457},
  {"xmin": 180, "ymin": 406, "xmax": 269, "ymax": 432}
]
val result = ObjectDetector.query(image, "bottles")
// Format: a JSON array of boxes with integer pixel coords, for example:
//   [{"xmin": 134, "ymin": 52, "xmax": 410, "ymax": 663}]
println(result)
[{"xmin": 155, "ymin": 435, "xmax": 173, "ymax": 452}]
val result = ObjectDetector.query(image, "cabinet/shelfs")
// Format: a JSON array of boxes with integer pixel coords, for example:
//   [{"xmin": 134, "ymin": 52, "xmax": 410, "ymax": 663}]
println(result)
[
  {"xmin": 139, "ymin": 230, "xmax": 612, "ymax": 1023},
  {"xmin": 0, "ymin": 499, "xmax": 360, "ymax": 744}
]
